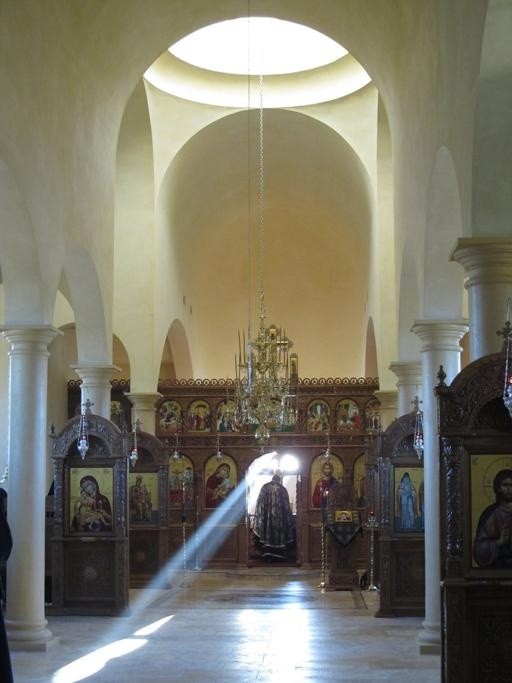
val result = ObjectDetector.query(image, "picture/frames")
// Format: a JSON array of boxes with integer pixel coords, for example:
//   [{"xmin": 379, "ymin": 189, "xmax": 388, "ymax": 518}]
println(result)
[
  {"xmin": 458, "ymin": 436, "xmax": 512, "ymax": 581},
  {"xmin": 62, "ymin": 456, "xmax": 117, "ymax": 536},
  {"xmin": 128, "ymin": 465, "xmax": 163, "ymax": 529},
  {"xmin": 386, "ymin": 461, "xmax": 423, "ymax": 535}
]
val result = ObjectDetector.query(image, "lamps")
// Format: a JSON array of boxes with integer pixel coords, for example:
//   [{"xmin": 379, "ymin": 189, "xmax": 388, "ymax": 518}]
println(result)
[
  {"xmin": 411, "ymin": 411, "xmax": 424, "ymax": 460},
  {"xmin": 502, "ymin": 298, "xmax": 512, "ymax": 419},
  {"xmin": 222, "ymin": 60, "xmax": 300, "ymax": 452},
  {"xmin": 129, "ymin": 431, "xmax": 138, "ymax": 467},
  {"xmin": 76, "ymin": 413, "xmax": 91, "ymax": 463}
]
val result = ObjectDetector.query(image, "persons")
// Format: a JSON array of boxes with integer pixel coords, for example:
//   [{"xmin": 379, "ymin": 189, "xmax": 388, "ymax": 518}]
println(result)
[
  {"xmin": 130, "ymin": 476, "xmax": 154, "ymax": 522},
  {"xmin": 210, "ymin": 473, "xmax": 233, "ymax": 501},
  {"xmin": 472, "ymin": 467, "xmax": 511, "ymax": 570},
  {"xmin": 245, "ymin": 474, "xmax": 297, "ymax": 560},
  {"xmin": 311, "ymin": 461, "xmax": 342, "ymax": 503},
  {"xmin": 204, "ymin": 462, "xmax": 235, "ymax": 508},
  {"xmin": 395, "ymin": 470, "xmax": 420, "ymax": 526},
  {"xmin": 70, "ymin": 474, "xmax": 113, "ymax": 530},
  {"xmin": 74, "ymin": 490, "xmax": 113, "ymax": 529}
]
